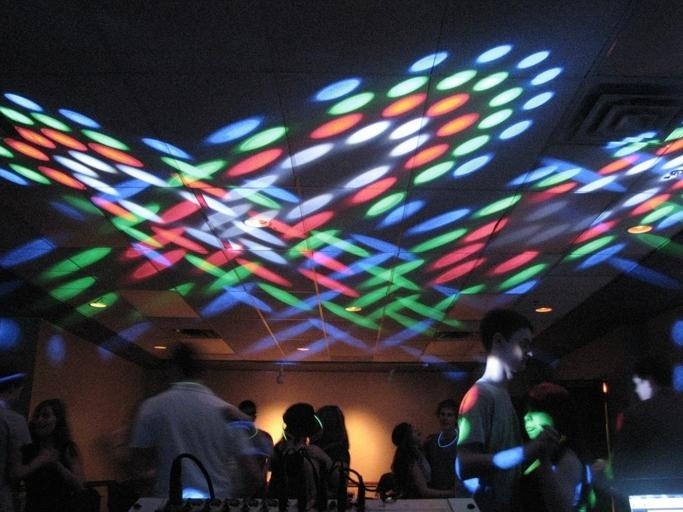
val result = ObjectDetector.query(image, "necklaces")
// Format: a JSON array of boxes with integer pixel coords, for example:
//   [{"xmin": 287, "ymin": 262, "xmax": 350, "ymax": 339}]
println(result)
[
  {"xmin": 247, "ymin": 427, "xmax": 257, "ymax": 440},
  {"xmin": 171, "ymin": 381, "xmax": 199, "ymax": 385},
  {"xmin": 436, "ymin": 428, "xmax": 459, "ymax": 448}
]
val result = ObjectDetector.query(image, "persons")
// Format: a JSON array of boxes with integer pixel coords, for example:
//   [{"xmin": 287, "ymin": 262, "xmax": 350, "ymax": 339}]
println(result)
[
  {"xmin": 238, "ymin": 398, "xmax": 272, "ymax": 499},
  {"xmin": 420, "ymin": 398, "xmax": 459, "ymax": 491},
  {"xmin": 112, "ymin": 339, "xmax": 260, "ymax": 500},
  {"xmin": 389, "ymin": 422, "xmax": 454, "ymax": 499},
  {"xmin": 21, "ymin": 398, "xmax": 84, "ymax": 511},
  {"xmin": 610, "ymin": 355, "xmax": 682, "ymax": 477},
  {"xmin": 0, "ymin": 356, "xmax": 54, "ymax": 512},
  {"xmin": 470, "ymin": 380, "xmax": 584, "ymax": 512},
  {"xmin": 454, "ymin": 307, "xmax": 562, "ymax": 511},
  {"xmin": 264, "ymin": 403, "xmax": 332, "ymax": 499},
  {"xmin": 310, "ymin": 406, "xmax": 350, "ymax": 500}
]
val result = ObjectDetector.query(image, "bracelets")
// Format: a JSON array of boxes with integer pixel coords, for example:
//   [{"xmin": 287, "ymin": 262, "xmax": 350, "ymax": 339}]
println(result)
[{"xmin": 27, "ymin": 463, "xmax": 33, "ymax": 473}]
